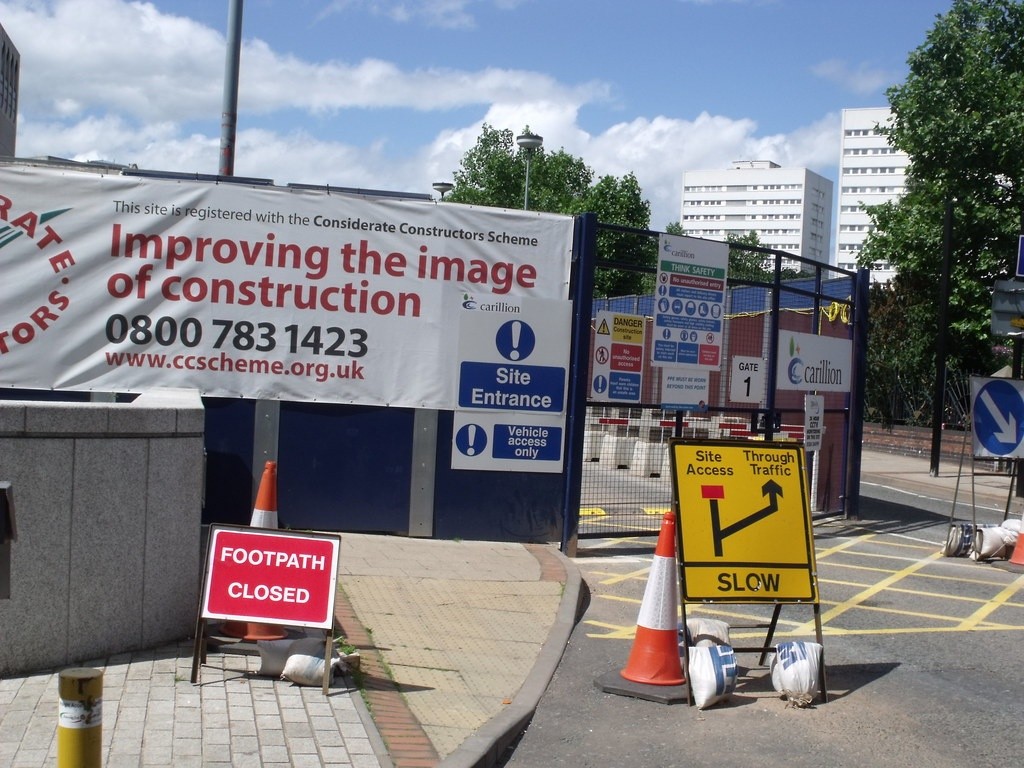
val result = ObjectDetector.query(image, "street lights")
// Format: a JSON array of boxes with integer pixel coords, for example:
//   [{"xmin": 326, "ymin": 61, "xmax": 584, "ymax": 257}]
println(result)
[{"xmin": 517, "ymin": 124, "xmax": 545, "ymax": 210}]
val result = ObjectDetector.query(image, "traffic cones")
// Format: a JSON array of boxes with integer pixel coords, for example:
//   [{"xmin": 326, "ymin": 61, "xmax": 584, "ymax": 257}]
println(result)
[
  {"xmin": 620, "ymin": 511, "xmax": 691, "ymax": 687},
  {"xmin": 211, "ymin": 460, "xmax": 293, "ymax": 642}
]
